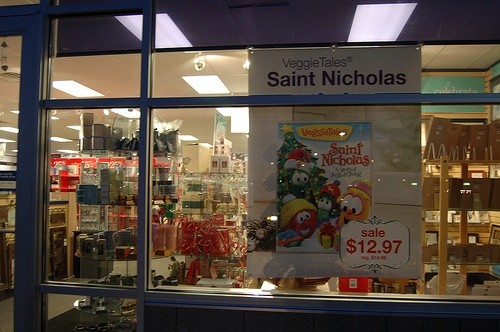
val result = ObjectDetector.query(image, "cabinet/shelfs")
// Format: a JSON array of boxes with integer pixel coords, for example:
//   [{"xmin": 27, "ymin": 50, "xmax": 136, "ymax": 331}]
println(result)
[
  {"xmin": 420, "ymin": 113, "xmax": 500, "ymax": 300},
  {"xmin": 72, "ymin": 110, "xmax": 182, "ymax": 332}
]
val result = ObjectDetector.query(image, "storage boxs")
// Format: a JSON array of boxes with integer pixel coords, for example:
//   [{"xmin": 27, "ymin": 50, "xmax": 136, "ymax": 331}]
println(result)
[
  {"xmin": 422, "ymin": 117, "xmax": 500, "ymax": 265},
  {"xmin": 183, "ymin": 143, "xmax": 254, "ymax": 289},
  {"xmin": 76, "ymin": 112, "xmax": 179, "ymax": 315}
]
